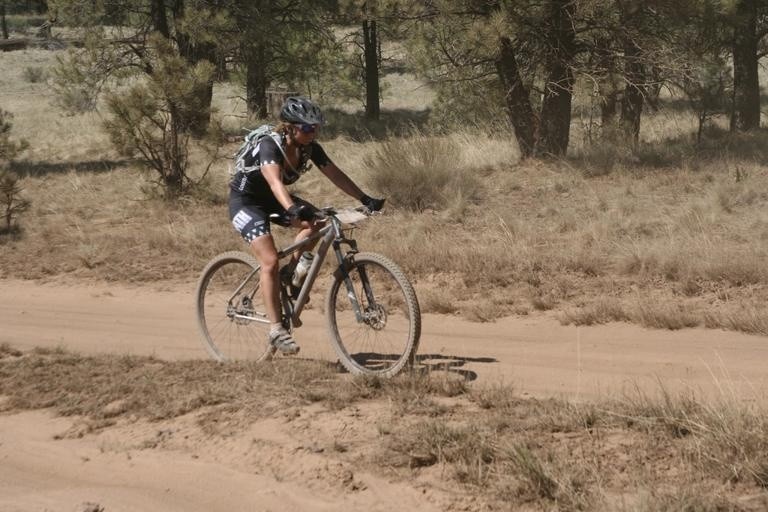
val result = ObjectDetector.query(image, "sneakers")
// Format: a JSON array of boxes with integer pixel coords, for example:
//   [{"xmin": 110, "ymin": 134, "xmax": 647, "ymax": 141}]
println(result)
[
  {"xmin": 268, "ymin": 324, "xmax": 301, "ymax": 356},
  {"xmin": 279, "ymin": 263, "xmax": 312, "ymax": 305}
]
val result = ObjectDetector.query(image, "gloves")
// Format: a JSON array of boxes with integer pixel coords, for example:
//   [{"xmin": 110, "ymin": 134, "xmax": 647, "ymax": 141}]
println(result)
[
  {"xmin": 360, "ymin": 193, "xmax": 385, "ymax": 211},
  {"xmin": 287, "ymin": 204, "xmax": 314, "ymax": 221}
]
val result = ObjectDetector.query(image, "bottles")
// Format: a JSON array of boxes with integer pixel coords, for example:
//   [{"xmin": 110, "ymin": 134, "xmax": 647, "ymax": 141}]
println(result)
[{"xmin": 292, "ymin": 251, "xmax": 313, "ymax": 288}]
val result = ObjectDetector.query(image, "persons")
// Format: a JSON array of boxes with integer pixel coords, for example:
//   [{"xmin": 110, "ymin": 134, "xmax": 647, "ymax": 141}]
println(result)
[{"xmin": 228, "ymin": 96, "xmax": 383, "ymax": 355}]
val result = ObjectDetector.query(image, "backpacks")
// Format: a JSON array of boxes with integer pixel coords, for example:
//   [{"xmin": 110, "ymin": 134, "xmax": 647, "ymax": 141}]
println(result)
[{"xmin": 233, "ymin": 122, "xmax": 283, "ymax": 168}]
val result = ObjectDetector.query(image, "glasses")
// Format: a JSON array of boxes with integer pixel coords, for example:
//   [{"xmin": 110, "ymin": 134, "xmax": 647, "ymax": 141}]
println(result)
[{"xmin": 294, "ymin": 122, "xmax": 317, "ymax": 134}]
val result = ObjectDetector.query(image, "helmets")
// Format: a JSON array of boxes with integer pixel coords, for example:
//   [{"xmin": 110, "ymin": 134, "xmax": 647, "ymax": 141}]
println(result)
[{"xmin": 279, "ymin": 96, "xmax": 325, "ymax": 126}]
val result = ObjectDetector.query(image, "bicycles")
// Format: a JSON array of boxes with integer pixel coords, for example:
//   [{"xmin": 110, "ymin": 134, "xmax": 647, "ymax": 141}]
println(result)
[{"xmin": 197, "ymin": 206, "xmax": 421, "ymax": 379}]
